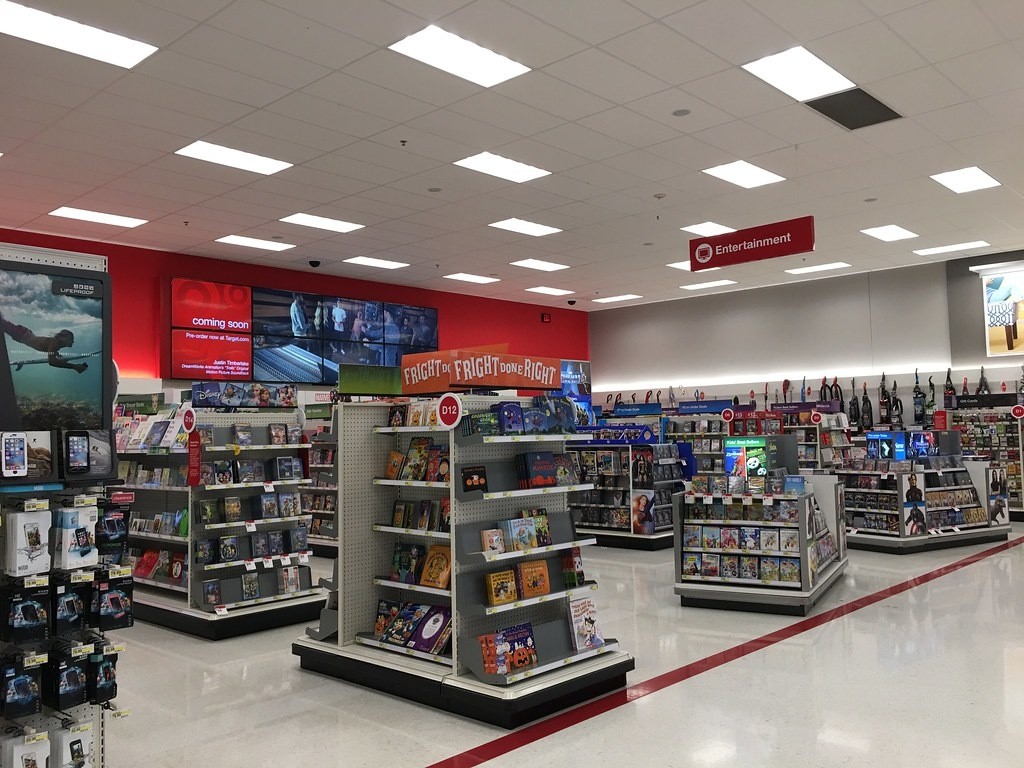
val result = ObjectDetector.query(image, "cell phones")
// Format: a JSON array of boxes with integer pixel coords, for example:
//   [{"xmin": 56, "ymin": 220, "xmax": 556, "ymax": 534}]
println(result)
[{"xmin": 0, "ymin": 431, "xmax": 127, "ymax": 768}]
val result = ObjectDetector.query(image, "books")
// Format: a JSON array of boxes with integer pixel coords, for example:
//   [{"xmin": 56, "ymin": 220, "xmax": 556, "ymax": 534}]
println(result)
[
  {"xmin": 681, "ymin": 497, "xmax": 803, "ymax": 588},
  {"xmin": 368, "ymin": 435, "xmax": 602, "ymax": 677}
]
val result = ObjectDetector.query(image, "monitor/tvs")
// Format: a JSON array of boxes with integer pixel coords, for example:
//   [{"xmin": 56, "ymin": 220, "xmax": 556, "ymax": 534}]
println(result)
[{"xmin": 169, "ymin": 275, "xmax": 438, "ymax": 386}]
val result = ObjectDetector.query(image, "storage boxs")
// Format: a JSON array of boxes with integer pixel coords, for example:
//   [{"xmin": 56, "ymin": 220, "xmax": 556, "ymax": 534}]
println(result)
[
  {"xmin": 0, "ymin": 502, "xmax": 134, "ymax": 768},
  {"xmin": 568, "ymin": 450, "xmax": 630, "ymax": 476}
]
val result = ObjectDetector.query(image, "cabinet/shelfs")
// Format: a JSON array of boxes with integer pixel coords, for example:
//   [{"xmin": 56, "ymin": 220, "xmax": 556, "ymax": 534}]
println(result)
[
  {"xmin": 665, "ymin": 416, "xmax": 730, "ymax": 474},
  {"xmin": 336, "ymin": 399, "xmax": 452, "ymax": 710},
  {"xmin": 179, "ymin": 405, "xmax": 328, "ymax": 642},
  {"xmin": 681, "ymin": 491, "xmax": 818, "ymax": 617},
  {"xmin": 116, "ymin": 448, "xmax": 188, "ymax": 630},
  {"xmin": 834, "ymin": 469, "xmax": 901, "ymax": 555},
  {"xmin": 439, "ymin": 395, "xmax": 635, "ymax": 731},
  {"xmin": 925, "ymin": 468, "xmax": 1012, "ymax": 552},
  {"xmin": 783, "ymin": 414, "xmax": 855, "ymax": 470},
  {"xmin": 303, "ymin": 421, "xmax": 338, "ymax": 560}
]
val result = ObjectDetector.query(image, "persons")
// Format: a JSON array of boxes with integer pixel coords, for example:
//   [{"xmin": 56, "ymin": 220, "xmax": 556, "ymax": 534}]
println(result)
[
  {"xmin": 632, "ymin": 453, "xmax": 654, "ymax": 488},
  {"xmin": 633, "ymin": 494, "xmax": 655, "ymax": 534},
  {"xmin": 411, "ymin": 314, "xmax": 432, "ymax": 352},
  {"xmin": 0, "ymin": 317, "xmax": 88, "ymax": 374},
  {"xmin": 361, "ymin": 303, "xmax": 401, "ymax": 367},
  {"xmin": 989, "ymin": 469, "xmax": 1007, "ymax": 495},
  {"xmin": 397, "ymin": 317, "xmax": 412, "ymax": 367},
  {"xmin": 349, "ymin": 311, "xmax": 364, "ymax": 355},
  {"xmin": 332, "ymin": 298, "xmax": 347, "ymax": 355},
  {"xmin": 905, "ymin": 474, "xmax": 924, "ymax": 503},
  {"xmin": 251, "ymin": 293, "xmax": 333, "ymax": 353}
]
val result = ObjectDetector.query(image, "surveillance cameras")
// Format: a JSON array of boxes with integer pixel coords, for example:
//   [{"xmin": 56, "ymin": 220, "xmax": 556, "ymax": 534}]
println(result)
[
  {"xmin": 568, "ymin": 301, "xmax": 576, "ymax": 305},
  {"xmin": 309, "ymin": 261, "xmax": 320, "ymax": 267}
]
